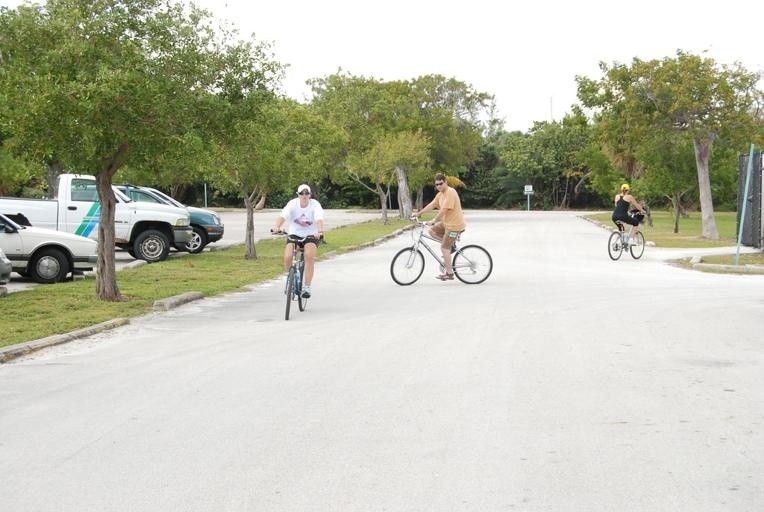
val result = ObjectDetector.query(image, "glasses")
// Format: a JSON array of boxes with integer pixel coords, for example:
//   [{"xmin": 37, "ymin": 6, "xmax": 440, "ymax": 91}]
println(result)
[
  {"xmin": 435, "ymin": 182, "xmax": 443, "ymax": 186},
  {"xmin": 301, "ymin": 191, "xmax": 309, "ymax": 196}
]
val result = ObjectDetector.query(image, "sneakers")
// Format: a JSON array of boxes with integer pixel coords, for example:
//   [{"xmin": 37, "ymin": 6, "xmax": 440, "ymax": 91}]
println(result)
[{"xmin": 302, "ymin": 291, "xmax": 311, "ymax": 298}]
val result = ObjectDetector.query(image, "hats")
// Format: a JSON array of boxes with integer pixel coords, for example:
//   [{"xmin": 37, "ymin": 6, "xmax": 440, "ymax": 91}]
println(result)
[
  {"xmin": 298, "ymin": 184, "xmax": 311, "ymax": 194},
  {"xmin": 621, "ymin": 184, "xmax": 629, "ymax": 190}
]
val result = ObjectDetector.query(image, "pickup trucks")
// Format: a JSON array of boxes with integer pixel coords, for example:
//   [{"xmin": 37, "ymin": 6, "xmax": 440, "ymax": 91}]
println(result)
[{"xmin": 0, "ymin": 169, "xmax": 191, "ymax": 265}]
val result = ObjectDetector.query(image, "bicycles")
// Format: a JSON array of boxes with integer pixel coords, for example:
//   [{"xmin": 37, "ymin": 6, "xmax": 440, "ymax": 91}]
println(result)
[
  {"xmin": 269, "ymin": 229, "xmax": 328, "ymax": 321},
  {"xmin": 607, "ymin": 209, "xmax": 647, "ymax": 260},
  {"xmin": 389, "ymin": 214, "xmax": 495, "ymax": 284}
]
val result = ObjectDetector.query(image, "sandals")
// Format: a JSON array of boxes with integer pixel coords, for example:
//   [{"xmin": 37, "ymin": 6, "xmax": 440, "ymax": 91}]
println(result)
[{"xmin": 436, "ymin": 272, "xmax": 454, "ymax": 280}]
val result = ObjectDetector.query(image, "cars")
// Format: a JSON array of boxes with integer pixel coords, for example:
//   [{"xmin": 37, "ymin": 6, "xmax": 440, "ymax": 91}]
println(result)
[
  {"xmin": 0, "ymin": 214, "xmax": 97, "ymax": 287},
  {"xmin": 140, "ymin": 185, "xmax": 183, "ymax": 207},
  {"xmin": 0, "ymin": 248, "xmax": 14, "ymax": 288}
]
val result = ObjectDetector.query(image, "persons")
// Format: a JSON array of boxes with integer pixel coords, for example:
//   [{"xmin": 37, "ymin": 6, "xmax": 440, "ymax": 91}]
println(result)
[
  {"xmin": 273, "ymin": 184, "xmax": 324, "ymax": 298},
  {"xmin": 413, "ymin": 173, "xmax": 464, "ymax": 280},
  {"xmin": 612, "ymin": 184, "xmax": 646, "ymax": 246}
]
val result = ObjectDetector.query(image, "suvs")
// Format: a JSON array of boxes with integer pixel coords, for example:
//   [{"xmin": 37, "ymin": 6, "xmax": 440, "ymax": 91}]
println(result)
[{"xmin": 73, "ymin": 183, "xmax": 226, "ymax": 255}]
